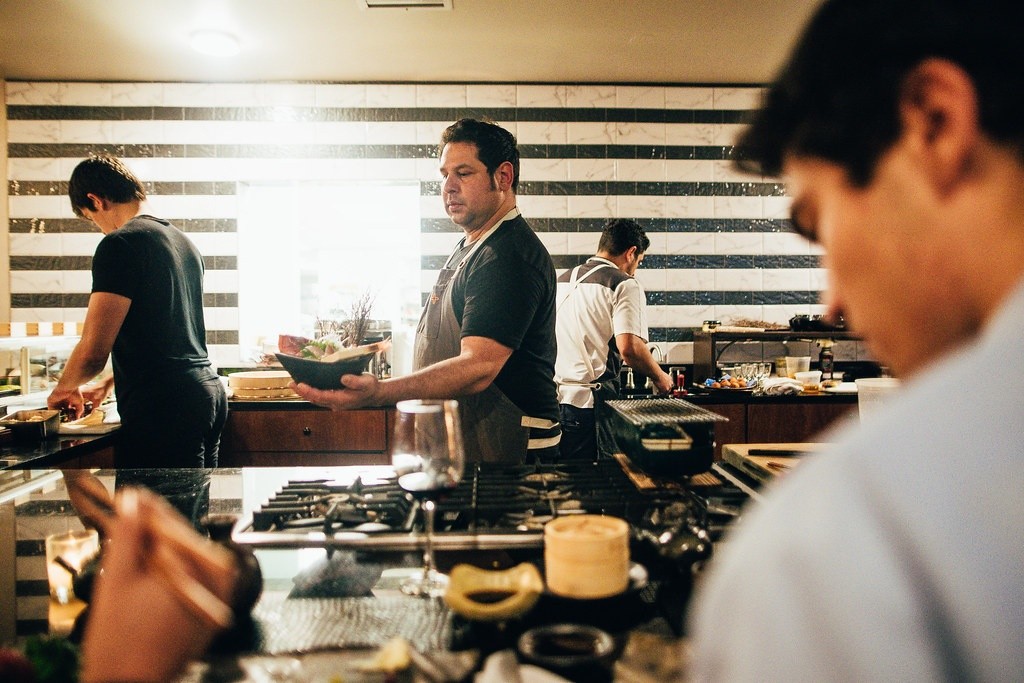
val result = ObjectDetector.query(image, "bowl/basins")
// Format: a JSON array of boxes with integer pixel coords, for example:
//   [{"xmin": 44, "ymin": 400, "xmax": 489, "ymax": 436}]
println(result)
[
  {"xmin": 228, "ymin": 372, "xmax": 293, "ymax": 400},
  {"xmin": 277, "ymin": 344, "xmax": 372, "ymax": 390}
]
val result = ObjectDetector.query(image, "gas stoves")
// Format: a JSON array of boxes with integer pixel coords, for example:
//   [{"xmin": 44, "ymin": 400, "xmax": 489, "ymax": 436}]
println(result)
[{"xmin": 233, "ymin": 461, "xmax": 591, "ymax": 553}]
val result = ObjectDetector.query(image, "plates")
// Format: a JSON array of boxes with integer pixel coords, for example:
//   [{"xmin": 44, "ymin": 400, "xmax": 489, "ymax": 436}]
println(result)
[
  {"xmin": 704, "ymin": 379, "xmax": 756, "ymax": 393},
  {"xmin": 442, "ymin": 563, "xmax": 542, "ymax": 620}
]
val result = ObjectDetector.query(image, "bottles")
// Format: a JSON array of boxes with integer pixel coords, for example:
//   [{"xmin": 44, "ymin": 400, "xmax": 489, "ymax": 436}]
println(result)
[{"xmin": 819, "ymin": 343, "xmax": 834, "ymax": 388}]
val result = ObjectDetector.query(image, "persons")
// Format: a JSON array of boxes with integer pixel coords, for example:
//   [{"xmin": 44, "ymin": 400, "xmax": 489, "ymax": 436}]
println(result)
[
  {"xmin": 550, "ymin": 217, "xmax": 675, "ymax": 462},
  {"xmin": 78, "ymin": 484, "xmax": 237, "ymax": 683},
  {"xmin": 46, "ymin": 156, "xmax": 228, "ymax": 469},
  {"xmin": 682, "ymin": 0, "xmax": 1024, "ymax": 683},
  {"xmin": 288, "ymin": 117, "xmax": 563, "ymax": 466}
]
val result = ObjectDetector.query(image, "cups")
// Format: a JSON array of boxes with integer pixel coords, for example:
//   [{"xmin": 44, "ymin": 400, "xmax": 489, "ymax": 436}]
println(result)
[
  {"xmin": 795, "ymin": 369, "xmax": 821, "ymax": 393},
  {"xmin": 519, "ymin": 624, "xmax": 615, "ymax": 683},
  {"xmin": 785, "ymin": 355, "xmax": 810, "ymax": 379},
  {"xmin": 44, "ymin": 530, "xmax": 99, "ymax": 630},
  {"xmin": 856, "ymin": 378, "xmax": 899, "ymax": 423}
]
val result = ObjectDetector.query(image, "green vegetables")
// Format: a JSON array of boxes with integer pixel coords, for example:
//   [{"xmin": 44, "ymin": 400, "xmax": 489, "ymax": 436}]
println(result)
[{"xmin": 300, "ymin": 340, "xmax": 336, "ymax": 359}]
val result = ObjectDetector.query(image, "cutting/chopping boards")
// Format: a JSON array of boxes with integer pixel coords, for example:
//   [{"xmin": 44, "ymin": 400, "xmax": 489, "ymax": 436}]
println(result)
[
  {"xmin": 721, "ymin": 440, "xmax": 830, "ymax": 494},
  {"xmin": 58, "ymin": 415, "xmax": 121, "ymax": 434}
]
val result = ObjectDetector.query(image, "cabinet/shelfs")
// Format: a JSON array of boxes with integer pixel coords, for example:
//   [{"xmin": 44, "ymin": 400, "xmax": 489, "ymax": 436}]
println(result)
[
  {"xmin": 220, "ymin": 409, "xmax": 395, "ymax": 466},
  {"xmin": 709, "ymin": 402, "xmax": 854, "ymax": 460}
]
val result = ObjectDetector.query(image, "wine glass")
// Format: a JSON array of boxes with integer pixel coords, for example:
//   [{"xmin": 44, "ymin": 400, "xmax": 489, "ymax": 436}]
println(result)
[
  {"xmin": 391, "ymin": 400, "xmax": 460, "ymax": 597},
  {"xmin": 735, "ymin": 362, "xmax": 772, "ymax": 391}
]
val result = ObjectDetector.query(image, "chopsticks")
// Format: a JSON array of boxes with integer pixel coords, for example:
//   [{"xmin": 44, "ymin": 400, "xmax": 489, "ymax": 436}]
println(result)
[{"xmin": 68, "ymin": 478, "xmax": 233, "ymax": 628}]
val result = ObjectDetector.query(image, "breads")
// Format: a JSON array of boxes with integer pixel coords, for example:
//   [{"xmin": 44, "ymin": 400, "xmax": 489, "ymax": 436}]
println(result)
[
  {"xmin": 279, "ymin": 335, "xmax": 311, "ymax": 355},
  {"xmin": 320, "ymin": 342, "xmax": 382, "ymax": 362}
]
